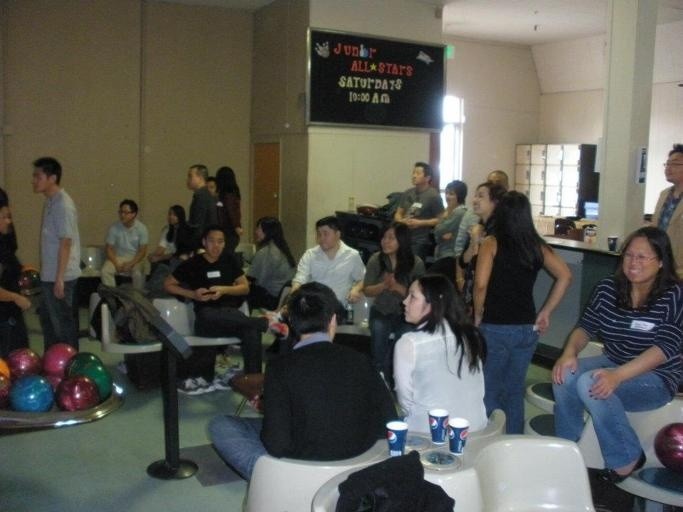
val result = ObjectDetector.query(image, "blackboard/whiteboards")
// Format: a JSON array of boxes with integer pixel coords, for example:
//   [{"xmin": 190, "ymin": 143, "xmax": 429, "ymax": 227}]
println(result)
[{"xmin": 307, "ymin": 26, "xmax": 447, "ymax": 132}]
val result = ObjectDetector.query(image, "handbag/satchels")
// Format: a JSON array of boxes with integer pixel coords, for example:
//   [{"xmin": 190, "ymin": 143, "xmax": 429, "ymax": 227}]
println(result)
[{"xmin": 335, "ymin": 450, "xmax": 455, "ymax": 511}]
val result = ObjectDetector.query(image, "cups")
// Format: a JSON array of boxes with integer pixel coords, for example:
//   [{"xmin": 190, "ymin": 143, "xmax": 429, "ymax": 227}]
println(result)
[
  {"xmin": 607, "ymin": 236, "xmax": 618, "ymax": 252},
  {"xmin": 448, "ymin": 417, "xmax": 470, "ymax": 456},
  {"xmin": 385, "ymin": 421, "xmax": 409, "ymax": 457},
  {"xmin": 428, "ymin": 409, "xmax": 450, "ymax": 445}
]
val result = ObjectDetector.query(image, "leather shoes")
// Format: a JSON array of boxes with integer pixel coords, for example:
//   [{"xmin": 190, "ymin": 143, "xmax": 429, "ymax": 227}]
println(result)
[{"xmin": 597, "ymin": 447, "xmax": 647, "ymax": 484}]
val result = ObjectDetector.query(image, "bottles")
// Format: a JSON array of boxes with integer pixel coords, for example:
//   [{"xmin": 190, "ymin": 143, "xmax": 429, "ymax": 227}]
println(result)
[{"xmin": 346, "ymin": 302, "xmax": 354, "ymax": 324}]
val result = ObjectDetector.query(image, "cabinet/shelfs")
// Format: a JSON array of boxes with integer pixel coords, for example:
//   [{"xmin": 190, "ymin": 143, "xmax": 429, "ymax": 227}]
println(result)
[{"xmin": 515, "ymin": 144, "xmax": 600, "ymax": 218}]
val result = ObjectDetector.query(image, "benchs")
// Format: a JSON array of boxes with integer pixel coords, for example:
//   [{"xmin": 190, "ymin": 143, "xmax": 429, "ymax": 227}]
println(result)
[
  {"xmin": 81, "ymin": 244, "xmax": 150, "ymax": 278},
  {"xmin": 278, "ymin": 285, "xmax": 395, "ymax": 340},
  {"xmin": 525, "ymin": 340, "xmax": 683, "ymax": 511},
  {"xmin": 243, "ymin": 409, "xmax": 508, "ymax": 512},
  {"xmin": 311, "ymin": 435, "xmax": 595, "ymax": 511},
  {"xmin": 102, "ymin": 297, "xmax": 249, "ymax": 354}
]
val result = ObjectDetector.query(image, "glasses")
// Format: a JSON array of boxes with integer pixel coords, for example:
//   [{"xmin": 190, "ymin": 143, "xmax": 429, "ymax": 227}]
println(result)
[
  {"xmin": 119, "ymin": 211, "xmax": 134, "ymax": 214},
  {"xmin": 663, "ymin": 163, "xmax": 683, "ymax": 166},
  {"xmin": 621, "ymin": 252, "xmax": 659, "ymax": 263}
]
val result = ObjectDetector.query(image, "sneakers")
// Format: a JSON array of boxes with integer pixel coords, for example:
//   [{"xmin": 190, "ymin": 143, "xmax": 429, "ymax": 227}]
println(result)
[
  {"xmin": 213, "ymin": 367, "xmax": 242, "ymax": 390},
  {"xmin": 195, "ymin": 377, "xmax": 216, "ymax": 393},
  {"xmin": 249, "ymin": 395, "xmax": 264, "ymax": 412},
  {"xmin": 259, "ymin": 314, "xmax": 288, "ymax": 340},
  {"xmin": 176, "ymin": 377, "xmax": 204, "ymax": 396}
]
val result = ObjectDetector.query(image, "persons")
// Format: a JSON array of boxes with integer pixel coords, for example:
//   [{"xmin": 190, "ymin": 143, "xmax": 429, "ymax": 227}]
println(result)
[
  {"xmin": 473, "ymin": 190, "xmax": 571, "ymax": 435},
  {"xmin": 205, "ymin": 282, "xmax": 388, "ymax": 483},
  {"xmin": 286, "ymin": 214, "xmax": 368, "ymax": 326},
  {"xmin": 241, "ymin": 217, "xmax": 296, "ymax": 313},
  {"xmin": 649, "ymin": 143, "xmax": 683, "ymax": 283},
  {"xmin": 100, "ymin": 199, "xmax": 152, "ymax": 290},
  {"xmin": 393, "ymin": 274, "xmax": 488, "ymax": 434},
  {"xmin": 551, "ymin": 228, "xmax": 682, "ymax": 497},
  {"xmin": 33, "ymin": 158, "xmax": 79, "ymax": 355},
  {"xmin": 167, "ymin": 164, "xmax": 262, "ymax": 397},
  {"xmin": 363, "ymin": 221, "xmax": 426, "ymax": 383},
  {"xmin": 393, "ymin": 161, "xmax": 507, "ymax": 328},
  {"xmin": 148, "ymin": 205, "xmax": 187, "ymax": 267},
  {"xmin": 0, "ymin": 199, "xmax": 29, "ymax": 363}
]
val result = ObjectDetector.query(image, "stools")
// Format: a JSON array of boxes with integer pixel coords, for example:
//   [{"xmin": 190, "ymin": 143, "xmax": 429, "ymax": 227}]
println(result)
[{"xmin": 229, "ymin": 371, "xmax": 264, "ymax": 417}]
